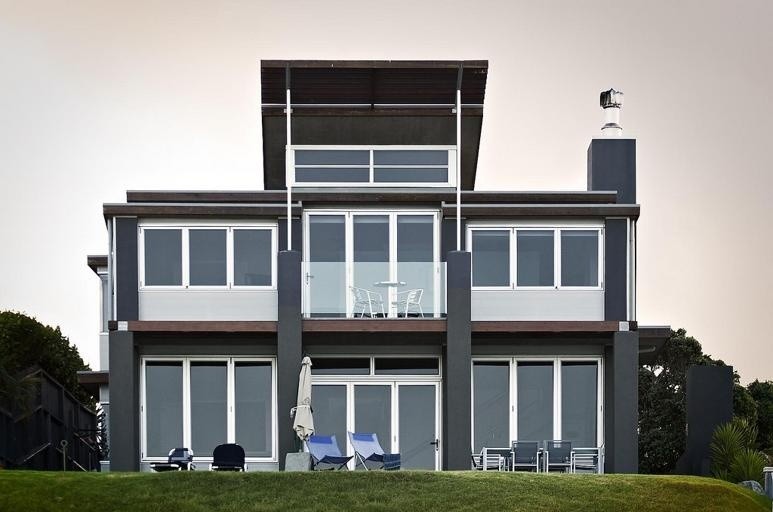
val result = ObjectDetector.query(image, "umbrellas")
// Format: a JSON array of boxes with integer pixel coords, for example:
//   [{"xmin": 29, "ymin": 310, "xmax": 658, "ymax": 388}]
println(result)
[{"xmin": 293, "ymin": 357, "xmax": 315, "ymax": 452}]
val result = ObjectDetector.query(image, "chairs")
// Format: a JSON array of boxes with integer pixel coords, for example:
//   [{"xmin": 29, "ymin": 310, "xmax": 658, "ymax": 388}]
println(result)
[
  {"xmin": 209, "ymin": 444, "xmax": 248, "ymax": 472},
  {"xmin": 149, "ymin": 447, "xmax": 197, "ymax": 473},
  {"xmin": 387, "ymin": 289, "xmax": 424, "ymax": 318},
  {"xmin": 348, "ymin": 432, "xmax": 401, "ymax": 470},
  {"xmin": 304, "ymin": 435, "xmax": 354, "ymax": 470},
  {"xmin": 472, "ymin": 440, "xmax": 606, "ymax": 473},
  {"xmin": 348, "ymin": 285, "xmax": 386, "ymax": 318}
]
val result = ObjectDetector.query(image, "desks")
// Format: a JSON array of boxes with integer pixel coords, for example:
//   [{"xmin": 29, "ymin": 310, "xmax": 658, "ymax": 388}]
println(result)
[{"xmin": 377, "ymin": 281, "xmax": 407, "ymax": 318}]
